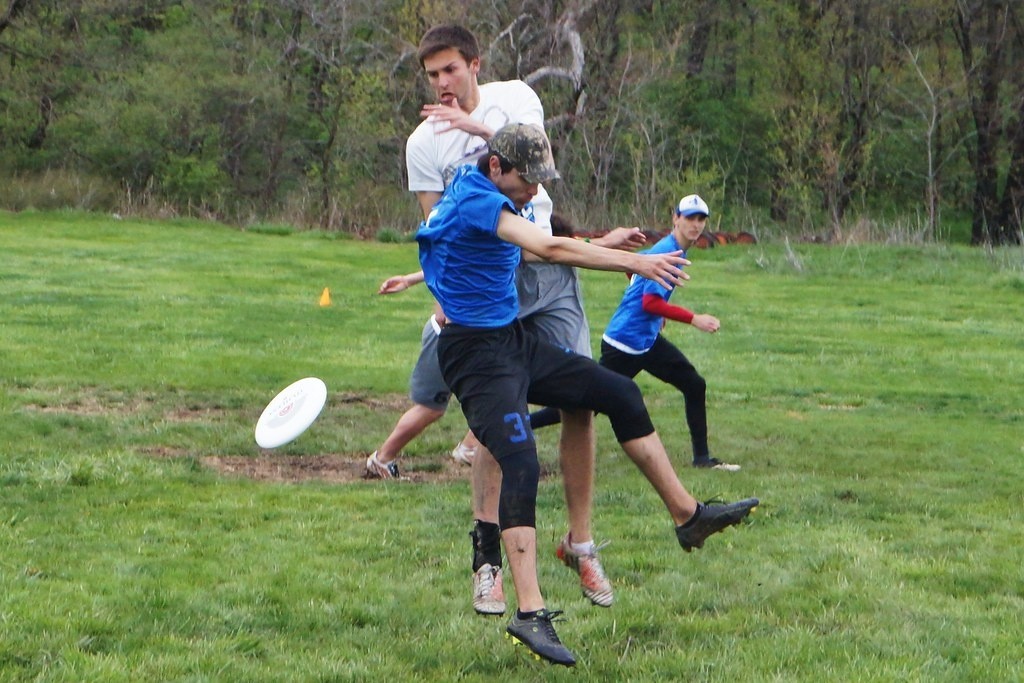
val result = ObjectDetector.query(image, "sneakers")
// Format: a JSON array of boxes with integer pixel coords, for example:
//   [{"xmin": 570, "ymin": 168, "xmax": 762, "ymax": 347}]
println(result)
[
  {"xmin": 450, "ymin": 442, "xmax": 476, "ymax": 467},
  {"xmin": 505, "ymin": 607, "xmax": 576, "ymax": 667},
  {"xmin": 674, "ymin": 494, "xmax": 759, "ymax": 552},
  {"xmin": 556, "ymin": 532, "xmax": 614, "ymax": 608},
  {"xmin": 472, "ymin": 562, "xmax": 507, "ymax": 617},
  {"xmin": 692, "ymin": 457, "xmax": 740, "ymax": 471},
  {"xmin": 365, "ymin": 450, "xmax": 409, "ymax": 483}
]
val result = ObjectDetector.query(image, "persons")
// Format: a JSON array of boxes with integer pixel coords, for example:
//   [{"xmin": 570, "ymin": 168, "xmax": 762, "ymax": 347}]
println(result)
[
  {"xmin": 531, "ymin": 194, "xmax": 741, "ymax": 474},
  {"xmin": 416, "ymin": 121, "xmax": 758, "ymax": 666},
  {"xmin": 366, "ymin": 213, "xmax": 574, "ymax": 483},
  {"xmin": 405, "ymin": 27, "xmax": 613, "ymax": 617}
]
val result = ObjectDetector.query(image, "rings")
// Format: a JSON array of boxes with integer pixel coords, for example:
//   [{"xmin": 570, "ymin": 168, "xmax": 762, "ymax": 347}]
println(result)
[{"xmin": 439, "ymin": 102, "xmax": 441, "ymax": 106}]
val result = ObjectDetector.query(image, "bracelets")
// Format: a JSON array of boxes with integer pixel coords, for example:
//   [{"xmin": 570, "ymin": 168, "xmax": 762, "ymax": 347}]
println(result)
[{"xmin": 584, "ymin": 237, "xmax": 590, "ymax": 243}]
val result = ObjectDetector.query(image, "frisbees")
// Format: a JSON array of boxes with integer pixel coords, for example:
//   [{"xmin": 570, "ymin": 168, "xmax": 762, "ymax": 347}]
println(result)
[{"xmin": 253, "ymin": 377, "xmax": 329, "ymax": 450}]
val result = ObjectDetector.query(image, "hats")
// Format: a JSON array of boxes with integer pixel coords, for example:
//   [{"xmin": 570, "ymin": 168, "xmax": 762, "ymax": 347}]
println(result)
[
  {"xmin": 488, "ymin": 122, "xmax": 561, "ymax": 184},
  {"xmin": 676, "ymin": 194, "xmax": 710, "ymax": 218}
]
